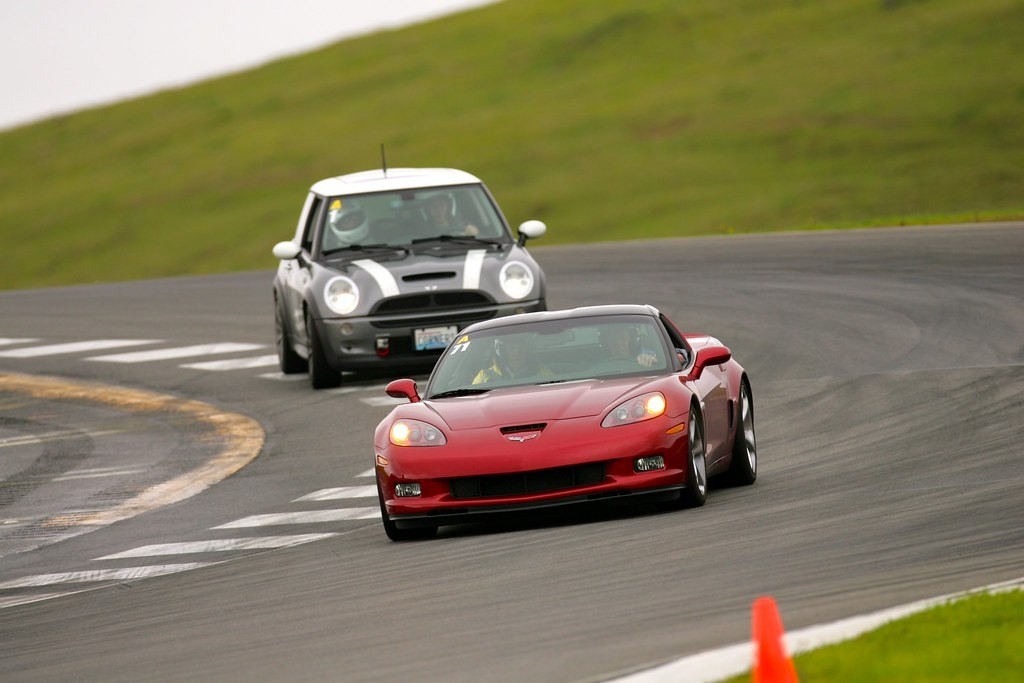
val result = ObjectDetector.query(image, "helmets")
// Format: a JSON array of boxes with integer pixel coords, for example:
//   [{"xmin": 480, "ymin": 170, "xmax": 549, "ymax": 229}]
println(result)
[
  {"xmin": 329, "ymin": 201, "xmax": 370, "ymax": 242},
  {"xmin": 417, "ymin": 190, "xmax": 456, "ymax": 221},
  {"xmin": 598, "ymin": 325, "xmax": 641, "ymax": 352},
  {"xmin": 495, "ymin": 337, "xmax": 529, "ymax": 356}
]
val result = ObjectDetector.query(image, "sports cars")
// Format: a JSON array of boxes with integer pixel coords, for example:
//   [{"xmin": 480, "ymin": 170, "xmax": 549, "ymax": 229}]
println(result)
[{"xmin": 374, "ymin": 303, "xmax": 759, "ymax": 541}]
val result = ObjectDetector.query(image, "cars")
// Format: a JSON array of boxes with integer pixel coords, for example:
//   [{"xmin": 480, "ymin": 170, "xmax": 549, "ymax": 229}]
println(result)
[{"xmin": 271, "ymin": 166, "xmax": 548, "ymax": 393}]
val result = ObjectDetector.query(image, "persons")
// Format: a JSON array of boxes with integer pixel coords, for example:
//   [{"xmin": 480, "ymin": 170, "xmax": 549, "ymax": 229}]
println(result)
[
  {"xmin": 599, "ymin": 323, "xmax": 688, "ymax": 370},
  {"xmin": 412, "ymin": 193, "xmax": 479, "ymax": 237},
  {"xmin": 323, "ymin": 200, "xmax": 388, "ymax": 250},
  {"xmin": 472, "ymin": 333, "xmax": 555, "ymax": 385}
]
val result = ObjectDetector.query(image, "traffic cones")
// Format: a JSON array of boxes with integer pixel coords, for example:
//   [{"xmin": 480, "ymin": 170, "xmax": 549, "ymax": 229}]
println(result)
[{"xmin": 751, "ymin": 599, "xmax": 799, "ymax": 683}]
